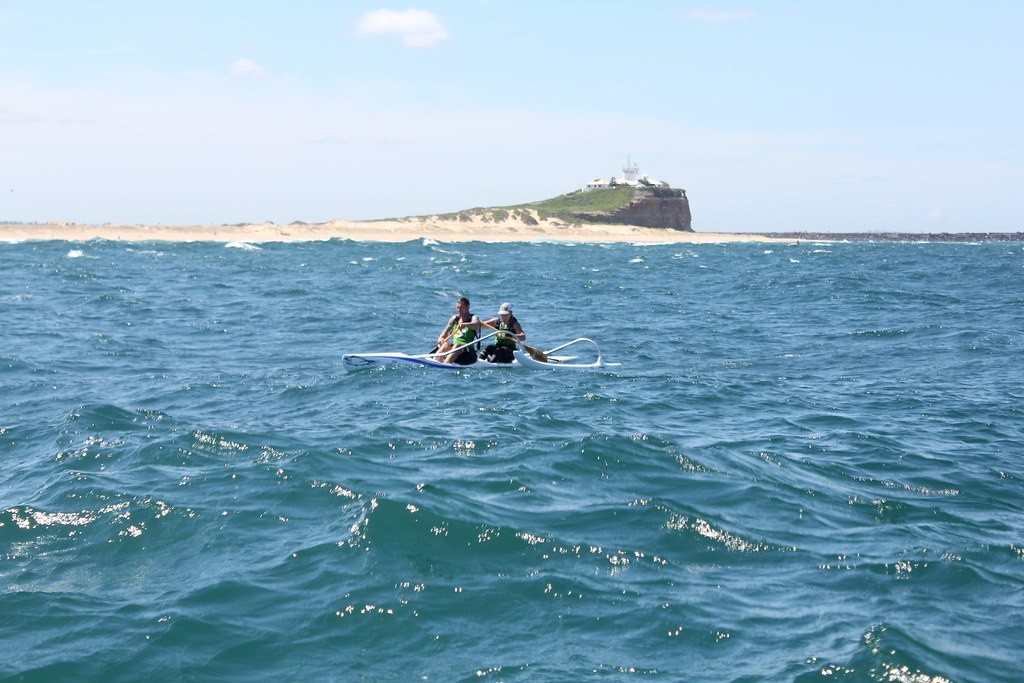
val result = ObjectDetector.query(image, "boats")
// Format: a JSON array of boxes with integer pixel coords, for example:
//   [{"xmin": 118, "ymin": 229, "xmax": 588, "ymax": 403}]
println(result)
[{"xmin": 340, "ymin": 330, "xmax": 622, "ymax": 376}]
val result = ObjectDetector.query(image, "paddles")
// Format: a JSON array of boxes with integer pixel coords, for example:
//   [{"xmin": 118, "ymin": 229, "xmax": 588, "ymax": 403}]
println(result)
[
  {"xmin": 477, "ymin": 319, "xmax": 548, "ymax": 363},
  {"xmin": 426, "ymin": 324, "xmax": 463, "ymax": 355}
]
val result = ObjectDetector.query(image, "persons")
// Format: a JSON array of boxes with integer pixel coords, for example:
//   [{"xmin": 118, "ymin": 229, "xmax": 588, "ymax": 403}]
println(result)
[
  {"xmin": 433, "ymin": 297, "xmax": 481, "ymax": 367},
  {"xmin": 479, "ymin": 302, "xmax": 527, "ymax": 364}
]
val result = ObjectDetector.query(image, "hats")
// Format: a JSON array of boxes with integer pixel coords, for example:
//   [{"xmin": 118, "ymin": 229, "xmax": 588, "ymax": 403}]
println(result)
[{"xmin": 497, "ymin": 303, "xmax": 513, "ymax": 316}]
